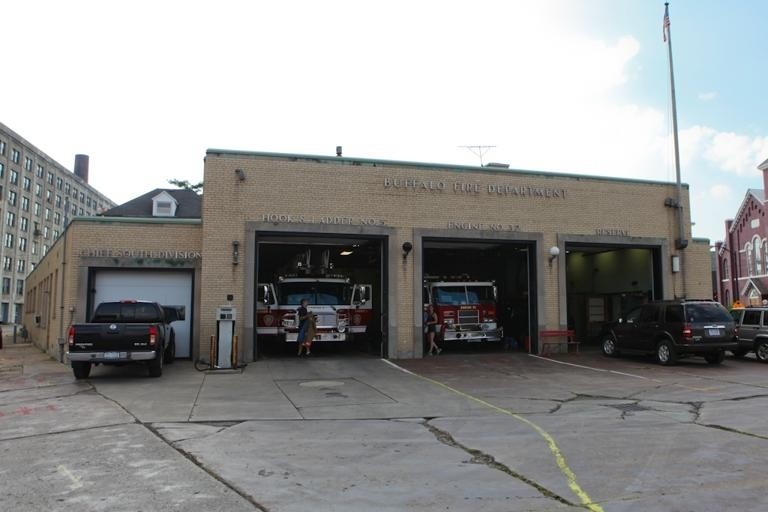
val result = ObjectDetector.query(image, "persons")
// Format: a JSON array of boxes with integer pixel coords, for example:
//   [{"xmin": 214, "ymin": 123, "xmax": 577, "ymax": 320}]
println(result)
[
  {"xmin": 296, "ymin": 299, "xmax": 316, "ymax": 357},
  {"xmin": 426, "ymin": 305, "xmax": 443, "ymax": 355}
]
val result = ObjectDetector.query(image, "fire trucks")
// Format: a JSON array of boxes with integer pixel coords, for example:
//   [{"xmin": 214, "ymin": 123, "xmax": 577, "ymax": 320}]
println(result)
[
  {"xmin": 422, "ymin": 270, "xmax": 506, "ymax": 343},
  {"xmin": 257, "ymin": 246, "xmax": 376, "ymax": 344}
]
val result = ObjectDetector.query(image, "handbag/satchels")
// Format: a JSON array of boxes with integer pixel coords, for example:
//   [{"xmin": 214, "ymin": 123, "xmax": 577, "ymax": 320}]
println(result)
[{"xmin": 424, "ymin": 326, "xmax": 428, "ymax": 333}]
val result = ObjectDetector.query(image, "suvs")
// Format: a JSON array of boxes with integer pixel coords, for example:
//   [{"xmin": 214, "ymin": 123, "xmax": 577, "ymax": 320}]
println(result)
[{"xmin": 727, "ymin": 305, "xmax": 768, "ymax": 363}]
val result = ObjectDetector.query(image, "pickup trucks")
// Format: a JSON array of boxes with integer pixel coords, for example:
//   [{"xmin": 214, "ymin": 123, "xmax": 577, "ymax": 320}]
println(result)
[{"xmin": 66, "ymin": 297, "xmax": 177, "ymax": 381}]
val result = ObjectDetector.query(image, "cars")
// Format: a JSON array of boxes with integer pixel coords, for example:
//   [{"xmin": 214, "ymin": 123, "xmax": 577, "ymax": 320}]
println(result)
[{"xmin": 602, "ymin": 298, "xmax": 740, "ymax": 364}]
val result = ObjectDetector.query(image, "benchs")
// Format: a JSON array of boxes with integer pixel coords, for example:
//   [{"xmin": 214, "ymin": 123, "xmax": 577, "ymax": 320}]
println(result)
[{"xmin": 539, "ymin": 329, "xmax": 581, "ymax": 356}]
